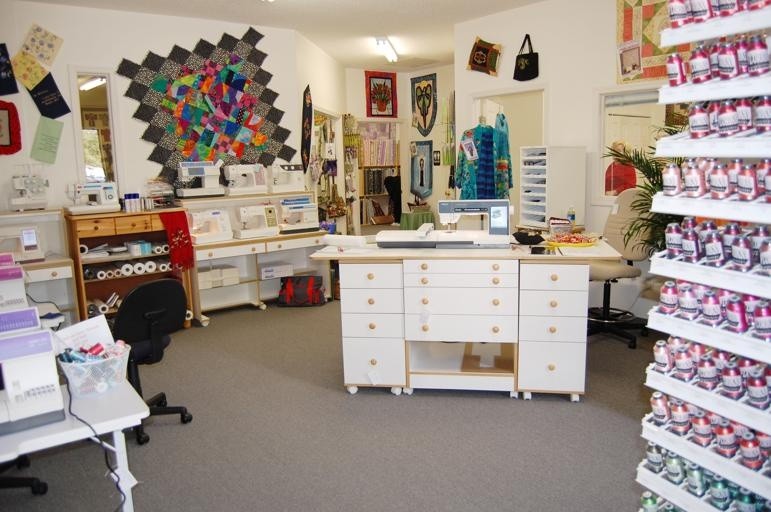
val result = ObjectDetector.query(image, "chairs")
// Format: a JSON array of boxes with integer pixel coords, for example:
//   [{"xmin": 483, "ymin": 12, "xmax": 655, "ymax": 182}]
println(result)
[
  {"xmin": 0, "ymin": 455, "xmax": 48, "ymax": 494},
  {"xmin": 103, "ymin": 279, "xmax": 192, "ymax": 446},
  {"xmin": 588, "ymin": 187, "xmax": 655, "ymax": 350}
]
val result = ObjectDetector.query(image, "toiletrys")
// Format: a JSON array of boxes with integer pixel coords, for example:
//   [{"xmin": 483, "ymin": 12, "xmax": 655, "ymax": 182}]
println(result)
[{"xmin": 57, "ymin": 340, "xmax": 127, "ymax": 393}]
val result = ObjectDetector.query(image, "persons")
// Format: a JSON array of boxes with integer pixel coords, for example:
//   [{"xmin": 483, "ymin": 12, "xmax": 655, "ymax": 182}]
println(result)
[
  {"xmin": 604, "ymin": 142, "xmax": 637, "ymax": 197},
  {"xmin": 419, "ymin": 157, "xmax": 425, "ymax": 187},
  {"xmin": 454, "ymin": 115, "xmax": 499, "ymax": 201}
]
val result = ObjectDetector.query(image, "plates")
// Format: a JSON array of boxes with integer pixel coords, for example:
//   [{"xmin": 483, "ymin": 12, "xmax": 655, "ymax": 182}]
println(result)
[{"xmin": 549, "ymin": 242, "xmax": 594, "ymax": 246}]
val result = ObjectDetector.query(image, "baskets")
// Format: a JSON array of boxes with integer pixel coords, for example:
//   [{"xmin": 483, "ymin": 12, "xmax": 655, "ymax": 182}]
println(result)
[{"xmin": 57, "ymin": 344, "xmax": 131, "ymax": 396}]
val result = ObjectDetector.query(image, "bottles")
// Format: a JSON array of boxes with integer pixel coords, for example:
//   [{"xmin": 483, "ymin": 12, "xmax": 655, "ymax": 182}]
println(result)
[
  {"xmin": 567, "ymin": 205, "xmax": 577, "ymax": 227},
  {"xmin": 125, "ymin": 193, "xmax": 141, "ymax": 213}
]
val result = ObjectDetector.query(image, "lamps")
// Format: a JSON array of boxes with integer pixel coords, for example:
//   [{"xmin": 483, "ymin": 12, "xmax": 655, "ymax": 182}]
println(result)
[
  {"xmin": 79, "ymin": 77, "xmax": 106, "ymax": 92},
  {"xmin": 375, "ymin": 37, "xmax": 398, "ymax": 63}
]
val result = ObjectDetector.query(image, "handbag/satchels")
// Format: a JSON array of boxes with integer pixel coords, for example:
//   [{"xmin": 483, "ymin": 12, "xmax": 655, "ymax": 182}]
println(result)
[
  {"xmin": 514, "ymin": 34, "xmax": 539, "ymax": 81},
  {"xmin": 344, "ymin": 152, "xmax": 353, "ymax": 173},
  {"xmin": 278, "ymin": 275, "xmax": 325, "ymax": 307},
  {"xmin": 327, "ymin": 184, "xmax": 347, "ymax": 218},
  {"xmin": 320, "ymin": 143, "xmax": 335, "ymax": 160}
]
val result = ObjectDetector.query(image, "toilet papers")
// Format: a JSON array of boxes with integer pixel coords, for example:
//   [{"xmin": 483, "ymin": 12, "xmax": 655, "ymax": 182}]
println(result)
[
  {"xmin": 161, "ymin": 243, "xmax": 170, "ymax": 253},
  {"xmin": 156, "ymin": 260, "xmax": 168, "ymax": 272},
  {"xmin": 114, "ymin": 268, "xmax": 121, "ymax": 278},
  {"xmin": 129, "ymin": 260, "xmax": 146, "ymax": 275},
  {"xmin": 152, "ymin": 244, "xmax": 163, "ymax": 254},
  {"xmin": 124, "ymin": 240, "xmax": 141, "ymax": 257},
  {"xmin": 113, "ymin": 262, "xmax": 133, "ymax": 276},
  {"xmin": 160, "ymin": 259, "xmax": 173, "ymax": 272},
  {"xmin": 94, "ymin": 299, "xmax": 109, "ymax": 314},
  {"xmin": 185, "ymin": 309, "xmax": 193, "ymax": 321},
  {"xmin": 133, "ymin": 239, "xmax": 152, "ymax": 256},
  {"xmin": 107, "ymin": 270, "xmax": 114, "ymax": 278},
  {"xmin": 96, "ymin": 270, "xmax": 106, "ymax": 281},
  {"xmin": 145, "ymin": 260, "xmax": 157, "ymax": 273},
  {"xmin": 80, "ymin": 245, "xmax": 87, "ymax": 255}
]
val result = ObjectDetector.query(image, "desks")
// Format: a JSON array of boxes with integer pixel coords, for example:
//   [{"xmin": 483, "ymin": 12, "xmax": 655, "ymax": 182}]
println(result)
[{"xmin": 0, "ymin": 379, "xmax": 151, "ymax": 512}]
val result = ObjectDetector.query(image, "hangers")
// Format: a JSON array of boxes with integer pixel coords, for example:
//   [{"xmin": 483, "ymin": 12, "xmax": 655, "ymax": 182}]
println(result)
[
  {"xmin": 496, "ymin": 109, "xmax": 504, "ymax": 120},
  {"xmin": 479, "ymin": 116, "xmax": 493, "ymax": 132}
]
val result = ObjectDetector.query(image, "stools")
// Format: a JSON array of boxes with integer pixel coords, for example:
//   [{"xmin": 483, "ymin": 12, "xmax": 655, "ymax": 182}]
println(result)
[{"xmin": 402, "ymin": 212, "xmax": 433, "ymax": 230}]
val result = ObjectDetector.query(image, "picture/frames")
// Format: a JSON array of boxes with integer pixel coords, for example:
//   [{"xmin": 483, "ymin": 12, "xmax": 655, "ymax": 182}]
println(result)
[{"xmin": 365, "ymin": 70, "xmax": 398, "ymax": 118}]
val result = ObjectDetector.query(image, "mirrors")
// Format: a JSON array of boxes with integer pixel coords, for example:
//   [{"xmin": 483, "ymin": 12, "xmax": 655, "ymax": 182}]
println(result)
[{"xmin": 69, "ymin": 62, "xmax": 119, "ymax": 185}]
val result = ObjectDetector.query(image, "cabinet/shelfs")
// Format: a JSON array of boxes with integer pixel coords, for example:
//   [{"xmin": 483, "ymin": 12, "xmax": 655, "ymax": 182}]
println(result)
[
  {"xmin": 518, "ymin": 146, "xmax": 586, "ymax": 226},
  {"xmin": 358, "ymin": 167, "xmax": 401, "ymax": 226},
  {"xmin": 178, "ymin": 194, "xmax": 331, "ymax": 326},
  {"xmin": 338, "ymin": 255, "xmax": 588, "ymax": 401},
  {"xmin": 65, "ymin": 209, "xmax": 202, "ymax": 332},
  {"xmin": 0, "ymin": 205, "xmax": 82, "ymax": 328},
  {"xmin": 637, "ymin": 0, "xmax": 771, "ymax": 512}
]
